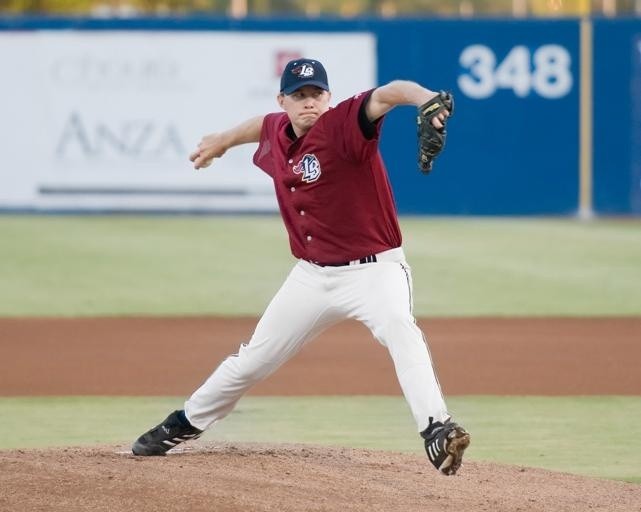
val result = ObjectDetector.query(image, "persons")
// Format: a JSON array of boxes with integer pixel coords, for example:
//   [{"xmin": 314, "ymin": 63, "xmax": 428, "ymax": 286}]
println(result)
[{"xmin": 132, "ymin": 59, "xmax": 471, "ymax": 476}]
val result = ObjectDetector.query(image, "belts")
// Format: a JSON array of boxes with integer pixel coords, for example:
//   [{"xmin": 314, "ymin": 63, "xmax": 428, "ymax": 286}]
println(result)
[{"xmin": 328, "ymin": 254, "xmax": 376, "ymax": 267}]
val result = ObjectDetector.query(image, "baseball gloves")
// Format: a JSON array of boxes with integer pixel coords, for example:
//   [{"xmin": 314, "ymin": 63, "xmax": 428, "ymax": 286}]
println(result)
[{"xmin": 417, "ymin": 90, "xmax": 455, "ymax": 175}]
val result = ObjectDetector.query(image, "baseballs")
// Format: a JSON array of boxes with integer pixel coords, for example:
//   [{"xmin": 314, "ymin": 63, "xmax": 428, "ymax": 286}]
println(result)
[{"xmin": 194, "ymin": 155, "xmax": 213, "ymax": 168}]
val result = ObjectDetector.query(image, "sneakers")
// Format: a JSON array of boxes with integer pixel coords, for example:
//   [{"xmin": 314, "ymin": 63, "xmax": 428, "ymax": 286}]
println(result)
[
  {"xmin": 132, "ymin": 410, "xmax": 202, "ymax": 456},
  {"xmin": 419, "ymin": 416, "xmax": 471, "ymax": 475}
]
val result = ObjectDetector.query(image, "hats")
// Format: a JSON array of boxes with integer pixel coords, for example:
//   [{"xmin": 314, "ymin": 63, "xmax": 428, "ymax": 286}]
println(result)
[{"xmin": 280, "ymin": 59, "xmax": 329, "ymax": 95}]
